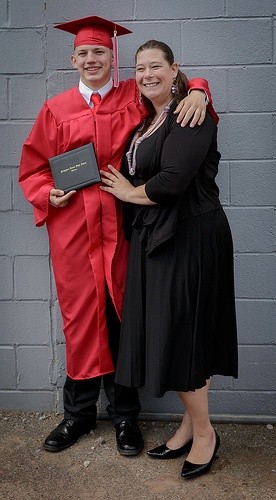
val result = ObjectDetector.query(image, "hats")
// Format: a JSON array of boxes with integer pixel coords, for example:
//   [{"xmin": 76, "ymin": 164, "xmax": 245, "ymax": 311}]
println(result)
[{"xmin": 54, "ymin": 15, "xmax": 133, "ymax": 87}]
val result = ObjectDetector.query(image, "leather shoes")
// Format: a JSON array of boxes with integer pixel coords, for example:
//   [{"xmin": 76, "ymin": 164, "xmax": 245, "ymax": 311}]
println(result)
[
  {"xmin": 43, "ymin": 418, "xmax": 84, "ymax": 451},
  {"xmin": 116, "ymin": 418, "xmax": 143, "ymax": 455}
]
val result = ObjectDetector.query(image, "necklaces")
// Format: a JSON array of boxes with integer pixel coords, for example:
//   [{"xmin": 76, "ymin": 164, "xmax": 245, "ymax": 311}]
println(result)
[{"xmin": 126, "ymin": 98, "xmax": 173, "ymax": 176}]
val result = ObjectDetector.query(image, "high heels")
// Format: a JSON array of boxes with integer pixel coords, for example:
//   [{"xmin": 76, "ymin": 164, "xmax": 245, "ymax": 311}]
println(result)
[
  {"xmin": 181, "ymin": 427, "xmax": 220, "ymax": 478},
  {"xmin": 147, "ymin": 438, "xmax": 193, "ymax": 459}
]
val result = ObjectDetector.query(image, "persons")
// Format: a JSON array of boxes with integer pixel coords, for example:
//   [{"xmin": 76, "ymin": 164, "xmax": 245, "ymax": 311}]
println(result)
[
  {"xmin": 17, "ymin": 15, "xmax": 220, "ymax": 457},
  {"xmin": 99, "ymin": 40, "xmax": 238, "ymax": 479}
]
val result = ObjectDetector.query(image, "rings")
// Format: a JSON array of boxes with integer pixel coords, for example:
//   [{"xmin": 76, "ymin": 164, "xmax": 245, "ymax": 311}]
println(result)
[{"xmin": 111, "ymin": 180, "xmax": 113, "ymax": 186}]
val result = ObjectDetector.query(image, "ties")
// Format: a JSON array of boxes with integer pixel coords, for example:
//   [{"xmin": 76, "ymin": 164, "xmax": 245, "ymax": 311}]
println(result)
[{"xmin": 91, "ymin": 94, "xmax": 101, "ymax": 118}]
